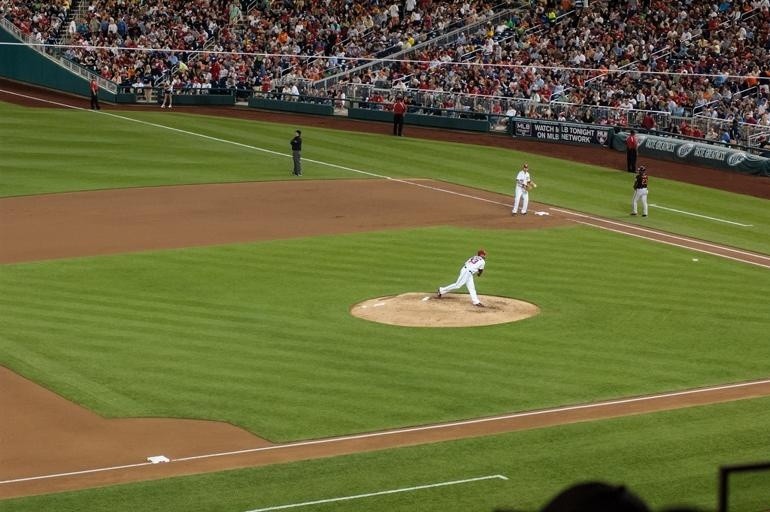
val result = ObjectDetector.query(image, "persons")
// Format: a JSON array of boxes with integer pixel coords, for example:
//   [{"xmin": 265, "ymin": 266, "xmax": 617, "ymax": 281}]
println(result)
[
  {"xmin": 510, "ymin": 164, "xmax": 535, "ymax": 217},
  {"xmin": 1, "ymin": 0, "xmax": 770, "ymax": 163},
  {"xmin": 436, "ymin": 247, "xmax": 489, "ymax": 307},
  {"xmin": 623, "ymin": 130, "xmax": 638, "ymax": 174},
  {"xmin": 289, "ymin": 130, "xmax": 304, "ymax": 178},
  {"xmin": 628, "ymin": 165, "xmax": 649, "ymax": 217}
]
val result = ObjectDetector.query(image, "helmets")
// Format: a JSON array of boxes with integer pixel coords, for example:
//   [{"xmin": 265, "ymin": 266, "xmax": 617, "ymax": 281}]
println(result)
[{"xmin": 637, "ymin": 166, "xmax": 646, "ymax": 173}]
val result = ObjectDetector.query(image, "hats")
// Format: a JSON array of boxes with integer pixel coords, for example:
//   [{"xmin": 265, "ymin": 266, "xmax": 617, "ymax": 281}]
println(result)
[
  {"xmin": 295, "ymin": 130, "xmax": 302, "ymax": 134},
  {"xmin": 524, "ymin": 164, "xmax": 528, "ymax": 168},
  {"xmin": 478, "ymin": 250, "xmax": 487, "ymax": 256}
]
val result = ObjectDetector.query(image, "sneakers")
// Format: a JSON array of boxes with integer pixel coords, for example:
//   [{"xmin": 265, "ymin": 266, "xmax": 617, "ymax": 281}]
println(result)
[
  {"xmin": 438, "ymin": 288, "xmax": 441, "ymax": 298},
  {"xmin": 512, "ymin": 212, "xmax": 528, "ymax": 216},
  {"xmin": 474, "ymin": 302, "xmax": 484, "ymax": 307},
  {"xmin": 161, "ymin": 104, "xmax": 172, "ymax": 108},
  {"xmin": 630, "ymin": 213, "xmax": 649, "ymax": 217},
  {"xmin": 292, "ymin": 172, "xmax": 302, "ymax": 176}
]
